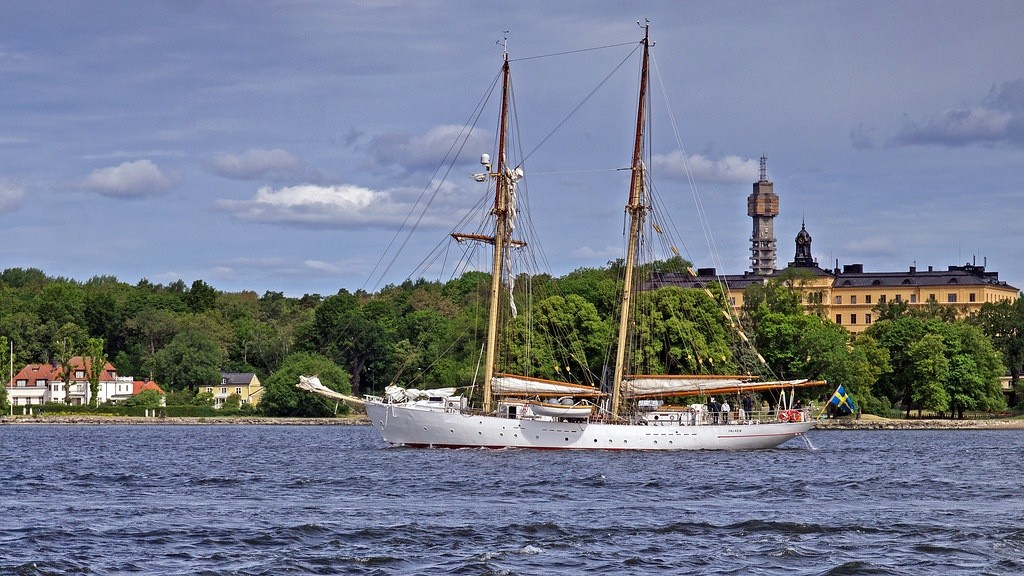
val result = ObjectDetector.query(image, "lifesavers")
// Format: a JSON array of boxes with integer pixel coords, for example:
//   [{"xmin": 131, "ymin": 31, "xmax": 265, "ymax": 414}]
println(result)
[{"xmin": 780, "ymin": 410, "xmax": 800, "ymax": 421}]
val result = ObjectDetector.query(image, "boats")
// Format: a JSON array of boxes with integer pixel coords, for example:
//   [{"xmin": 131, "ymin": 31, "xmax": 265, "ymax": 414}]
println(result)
[{"xmin": 528, "ymin": 399, "xmax": 593, "ymax": 419}]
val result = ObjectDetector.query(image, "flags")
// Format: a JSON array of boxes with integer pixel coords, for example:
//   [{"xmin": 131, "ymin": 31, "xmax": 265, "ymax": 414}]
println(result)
[{"xmin": 829, "ymin": 384, "xmax": 856, "ymax": 415}]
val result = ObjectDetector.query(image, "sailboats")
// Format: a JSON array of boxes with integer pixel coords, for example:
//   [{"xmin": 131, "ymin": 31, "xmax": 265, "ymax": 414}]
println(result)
[{"xmin": 294, "ymin": 19, "xmax": 830, "ymax": 454}]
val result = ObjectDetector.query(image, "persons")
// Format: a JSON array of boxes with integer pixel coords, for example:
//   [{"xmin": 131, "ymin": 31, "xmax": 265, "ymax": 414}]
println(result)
[
  {"xmin": 793, "ymin": 399, "xmax": 802, "ymax": 412},
  {"xmin": 711, "ymin": 396, "xmax": 752, "ymax": 425}
]
